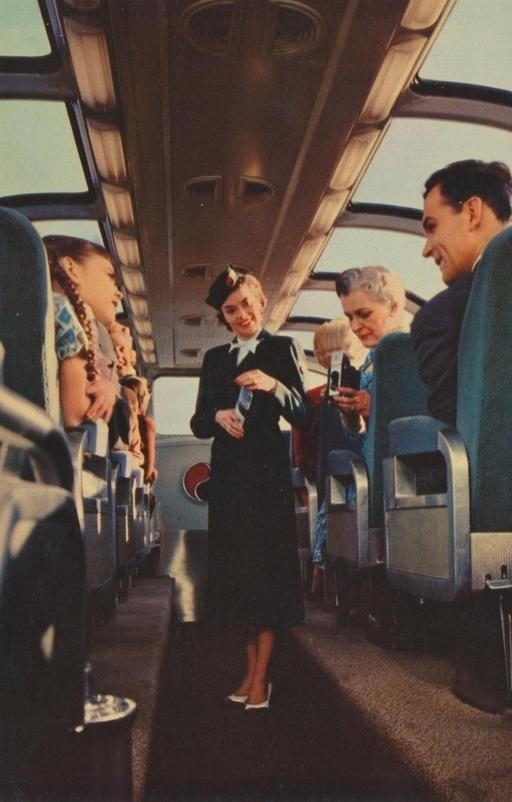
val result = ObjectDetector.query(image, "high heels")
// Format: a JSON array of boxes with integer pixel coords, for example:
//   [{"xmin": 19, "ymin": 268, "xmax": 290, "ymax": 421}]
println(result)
[
  {"xmin": 240, "ymin": 681, "xmax": 276, "ymax": 714},
  {"xmin": 224, "ymin": 685, "xmax": 250, "ymax": 705}
]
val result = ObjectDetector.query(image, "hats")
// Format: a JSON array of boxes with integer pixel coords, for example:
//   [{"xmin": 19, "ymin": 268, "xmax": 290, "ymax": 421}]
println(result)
[{"xmin": 204, "ymin": 264, "xmax": 238, "ymax": 311}]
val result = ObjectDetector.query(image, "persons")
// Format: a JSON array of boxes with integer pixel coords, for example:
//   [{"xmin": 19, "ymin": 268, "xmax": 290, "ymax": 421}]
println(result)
[
  {"xmin": 312, "ymin": 265, "xmax": 406, "ymax": 579},
  {"xmin": 290, "ymin": 318, "xmax": 366, "ymax": 508},
  {"xmin": 44, "ymin": 235, "xmax": 158, "ymax": 519},
  {"xmin": 409, "ymin": 160, "xmax": 511, "ymax": 492},
  {"xmin": 191, "ymin": 264, "xmax": 309, "ymax": 711}
]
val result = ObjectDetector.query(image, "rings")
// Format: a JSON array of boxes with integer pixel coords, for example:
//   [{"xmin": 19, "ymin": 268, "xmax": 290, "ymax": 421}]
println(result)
[{"xmin": 351, "ymin": 405, "xmax": 354, "ymax": 411}]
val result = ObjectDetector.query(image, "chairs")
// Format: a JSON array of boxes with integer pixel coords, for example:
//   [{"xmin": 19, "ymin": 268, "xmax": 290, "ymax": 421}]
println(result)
[
  {"xmin": 279, "ymin": 228, "xmax": 512, "ymax": 713},
  {"xmin": 0, "ymin": 207, "xmax": 158, "ymax": 801}
]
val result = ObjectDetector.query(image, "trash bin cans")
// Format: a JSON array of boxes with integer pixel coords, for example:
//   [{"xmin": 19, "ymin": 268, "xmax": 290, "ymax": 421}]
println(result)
[{"xmin": 159, "ymin": 527, "xmax": 209, "ymax": 624}]
